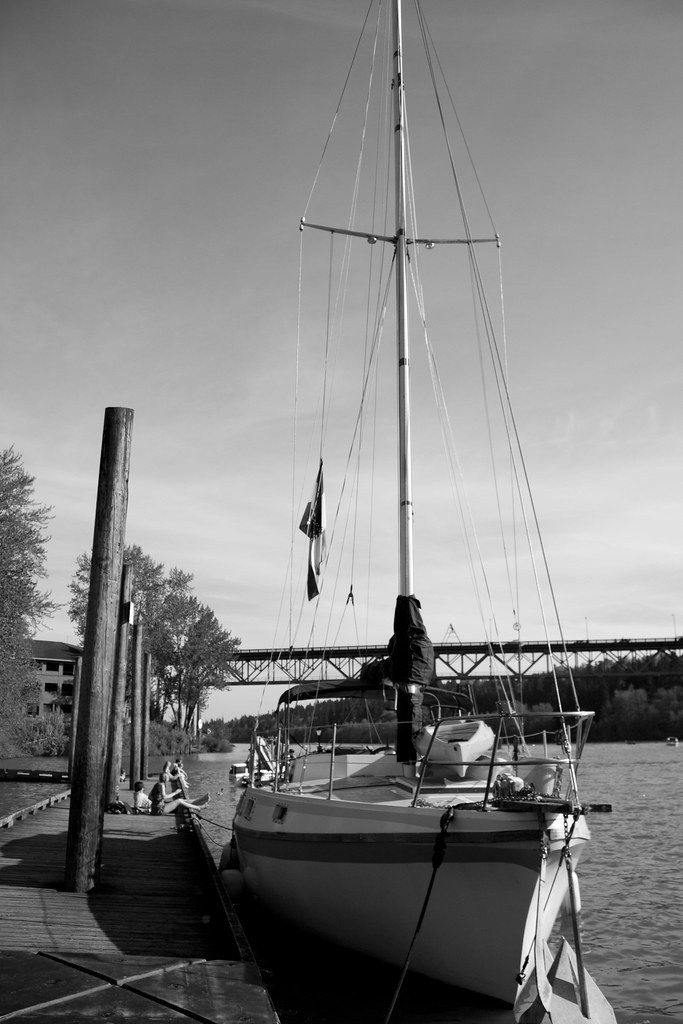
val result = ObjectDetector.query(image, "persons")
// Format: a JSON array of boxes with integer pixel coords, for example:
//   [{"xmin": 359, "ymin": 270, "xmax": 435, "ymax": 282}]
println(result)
[
  {"xmin": 133, "ymin": 782, "xmax": 152, "ymax": 813},
  {"xmin": 282, "ymin": 749, "xmax": 296, "ymax": 766},
  {"xmin": 148, "ymin": 772, "xmax": 210, "ymax": 816},
  {"xmin": 120, "ymin": 768, "xmax": 126, "ymax": 782},
  {"xmin": 163, "ymin": 759, "xmax": 191, "ymax": 789}
]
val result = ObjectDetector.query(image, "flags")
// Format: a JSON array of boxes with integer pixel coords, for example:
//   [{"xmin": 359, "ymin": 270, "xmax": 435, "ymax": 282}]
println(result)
[{"xmin": 299, "ymin": 459, "xmax": 328, "ymax": 600}]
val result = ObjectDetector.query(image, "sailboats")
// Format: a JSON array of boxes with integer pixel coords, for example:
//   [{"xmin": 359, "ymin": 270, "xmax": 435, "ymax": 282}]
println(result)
[{"xmin": 231, "ymin": 1, "xmax": 612, "ymax": 1023}]
